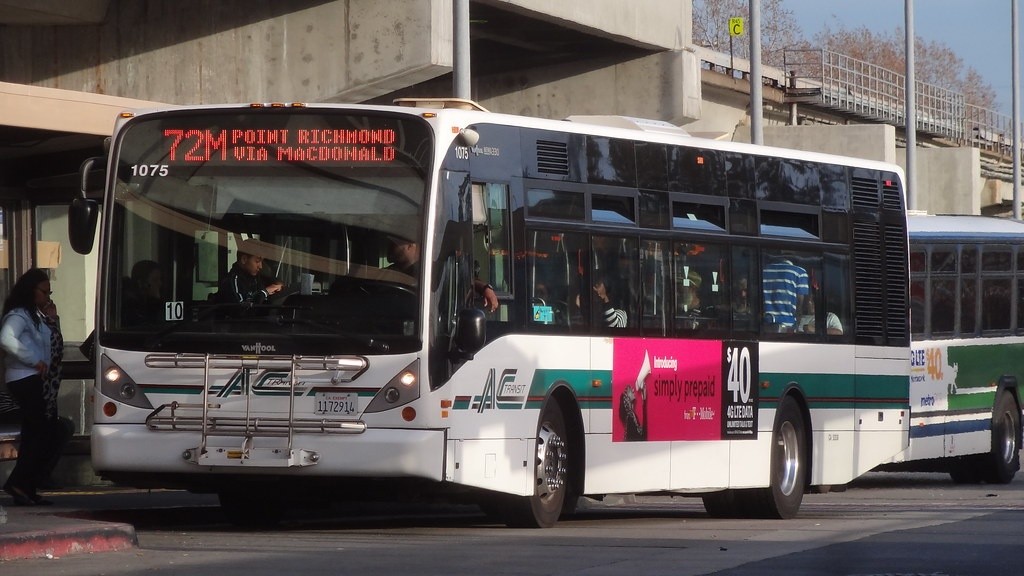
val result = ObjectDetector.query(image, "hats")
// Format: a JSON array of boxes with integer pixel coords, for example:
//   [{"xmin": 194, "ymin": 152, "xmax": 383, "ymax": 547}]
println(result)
[{"xmin": 677, "ymin": 271, "xmax": 702, "ymax": 288}]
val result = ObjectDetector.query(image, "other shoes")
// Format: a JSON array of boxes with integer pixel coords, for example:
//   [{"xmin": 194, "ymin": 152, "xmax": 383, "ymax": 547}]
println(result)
[{"xmin": 3, "ymin": 483, "xmax": 46, "ymax": 505}]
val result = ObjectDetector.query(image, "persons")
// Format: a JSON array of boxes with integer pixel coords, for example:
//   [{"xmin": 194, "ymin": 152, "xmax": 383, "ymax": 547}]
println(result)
[
  {"xmin": 527, "ymin": 243, "xmax": 844, "ymax": 336},
  {"xmin": 120, "ymin": 260, "xmax": 164, "ymax": 327},
  {"xmin": 350, "ymin": 217, "xmax": 500, "ymax": 312},
  {"xmin": 0, "ymin": 268, "xmax": 63, "ymax": 504},
  {"xmin": 219, "ymin": 239, "xmax": 286, "ymax": 316}
]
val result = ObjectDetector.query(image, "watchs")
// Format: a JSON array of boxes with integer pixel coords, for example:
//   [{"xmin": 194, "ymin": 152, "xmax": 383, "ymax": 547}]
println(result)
[{"xmin": 481, "ymin": 282, "xmax": 494, "ymax": 296}]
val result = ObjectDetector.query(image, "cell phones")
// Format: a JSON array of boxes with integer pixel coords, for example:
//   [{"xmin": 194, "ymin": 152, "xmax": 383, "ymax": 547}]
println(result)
[{"xmin": 42, "ymin": 299, "xmax": 51, "ymax": 311}]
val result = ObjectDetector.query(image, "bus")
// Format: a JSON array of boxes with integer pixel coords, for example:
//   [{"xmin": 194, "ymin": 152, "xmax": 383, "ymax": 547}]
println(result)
[
  {"xmin": 871, "ymin": 210, "xmax": 1024, "ymax": 484},
  {"xmin": 69, "ymin": 99, "xmax": 912, "ymax": 529}
]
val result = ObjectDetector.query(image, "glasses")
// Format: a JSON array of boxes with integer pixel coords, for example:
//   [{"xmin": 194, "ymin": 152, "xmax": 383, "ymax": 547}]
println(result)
[{"xmin": 34, "ymin": 289, "xmax": 53, "ymax": 296}]
